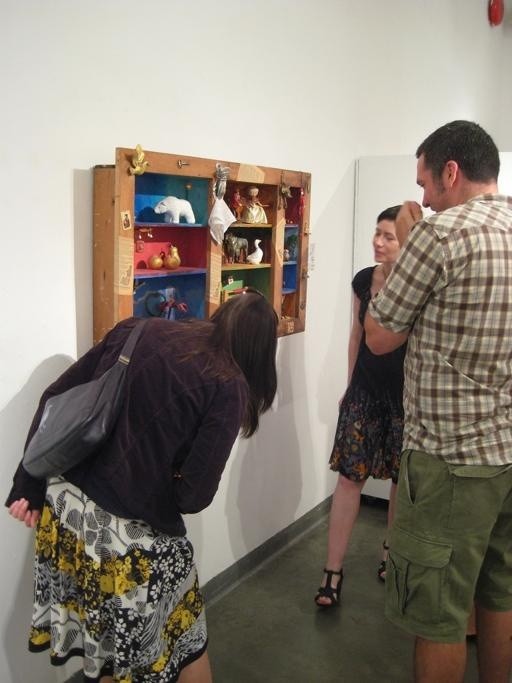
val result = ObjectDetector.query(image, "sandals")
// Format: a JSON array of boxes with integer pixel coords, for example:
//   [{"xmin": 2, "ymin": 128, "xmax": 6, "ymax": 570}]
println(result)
[
  {"xmin": 314, "ymin": 568, "xmax": 345, "ymax": 609},
  {"xmin": 378, "ymin": 538, "xmax": 387, "ymax": 582}
]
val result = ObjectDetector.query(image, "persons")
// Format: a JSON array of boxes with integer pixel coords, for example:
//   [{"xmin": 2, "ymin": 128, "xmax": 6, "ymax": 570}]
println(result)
[
  {"xmin": 364, "ymin": 120, "xmax": 511, "ymax": 682},
  {"xmin": 314, "ymin": 200, "xmax": 417, "ymax": 609},
  {"xmin": 5, "ymin": 288, "xmax": 281, "ymax": 681}
]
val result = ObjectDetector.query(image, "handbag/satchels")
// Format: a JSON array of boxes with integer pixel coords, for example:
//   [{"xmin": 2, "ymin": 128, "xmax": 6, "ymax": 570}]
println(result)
[{"xmin": 21, "ymin": 363, "xmax": 131, "ymax": 481}]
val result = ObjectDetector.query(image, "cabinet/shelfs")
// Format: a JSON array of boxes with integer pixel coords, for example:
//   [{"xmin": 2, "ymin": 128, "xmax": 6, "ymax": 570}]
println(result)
[{"xmin": 93, "ymin": 147, "xmax": 311, "ymax": 350}]
